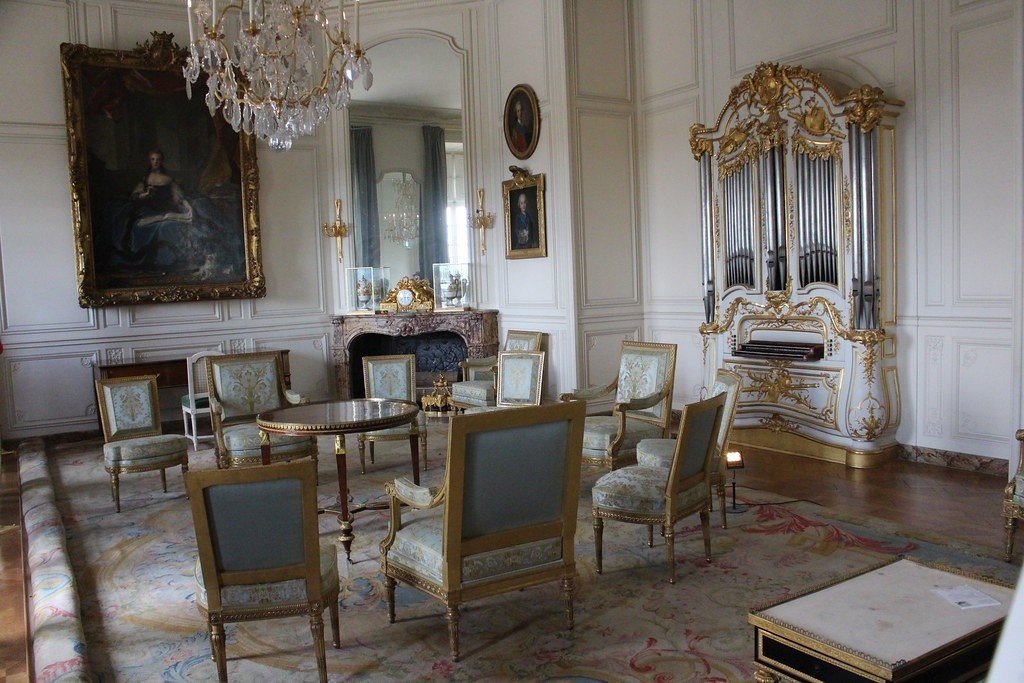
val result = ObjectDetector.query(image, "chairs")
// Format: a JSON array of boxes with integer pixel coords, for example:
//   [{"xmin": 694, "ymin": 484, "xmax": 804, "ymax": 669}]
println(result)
[
  {"xmin": 204, "ymin": 350, "xmax": 320, "ymax": 487},
  {"xmin": 184, "ymin": 455, "xmax": 340, "ymax": 683},
  {"xmin": 1000, "ymin": 428, "xmax": 1024, "ymax": 563},
  {"xmin": 591, "ymin": 390, "xmax": 727, "ymax": 585},
  {"xmin": 559, "ymin": 339, "xmax": 679, "ymax": 473},
  {"xmin": 636, "ymin": 368, "xmax": 744, "ymax": 530},
  {"xmin": 94, "ymin": 375, "xmax": 189, "ymax": 513},
  {"xmin": 181, "ymin": 351, "xmax": 226, "ymax": 452},
  {"xmin": 358, "ymin": 355, "xmax": 428, "ymax": 475},
  {"xmin": 378, "ymin": 400, "xmax": 588, "ymax": 663},
  {"xmin": 434, "ymin": 329, "xmax": 542, "ymax": 436}
]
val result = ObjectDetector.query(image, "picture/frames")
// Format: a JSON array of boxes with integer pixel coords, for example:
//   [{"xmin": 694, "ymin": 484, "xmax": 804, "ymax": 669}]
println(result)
[
  {"xmin": 496, "ymin": 350, "xmax": 545, "ymax": 407},
  {"xmin": 60, "ymin": 31, "xmax": 267, "ymax": 309},
  {"xmin": 503, "ymin": 84, "xmax": 540, "ymax": 160},
  {"xmin": 502, "ymin": 165, "xmax": 547, "ymax": 260}
]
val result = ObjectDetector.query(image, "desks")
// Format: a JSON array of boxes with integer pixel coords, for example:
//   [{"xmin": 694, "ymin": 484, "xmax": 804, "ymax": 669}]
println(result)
[
  {"xmin": 255, "ymin": 398, "xmax": 421, "ymax": 564},
  {"xmin": 90, "ymin": 346, "xmax": 289, "ymax": 439},
  {"xmin": 748, "ymin": 552, "xmax": 1018, "ymax": 683}
]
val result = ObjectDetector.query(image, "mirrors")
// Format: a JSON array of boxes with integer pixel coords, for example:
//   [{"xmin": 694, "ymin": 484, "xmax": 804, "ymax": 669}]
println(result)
[
  {"xmin": 347, "ymin": 36, "xmax": 472, "ymax": 310},
  {"xmin": 375, "ymin": 168, "xmax": 427, "ymax": 298}
]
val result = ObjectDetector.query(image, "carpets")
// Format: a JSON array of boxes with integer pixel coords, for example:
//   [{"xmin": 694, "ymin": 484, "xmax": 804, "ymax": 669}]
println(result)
[{"xmin": 16, "ymin": 418, "xmax": 1020, "ymax": 683}]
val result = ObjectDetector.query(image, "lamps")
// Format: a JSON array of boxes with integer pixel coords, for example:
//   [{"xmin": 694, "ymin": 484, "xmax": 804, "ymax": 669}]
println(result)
[
  {"xmin": 726, "ymin": 451, "xmax": 748, "ymax": 513},
  {"xmin": 182, "ymin": 0, "xmax": 374, "ymax": 150},
  {"xmin": 381, "ymin": 173, "xmax": 420, "ymax": 250}
]
